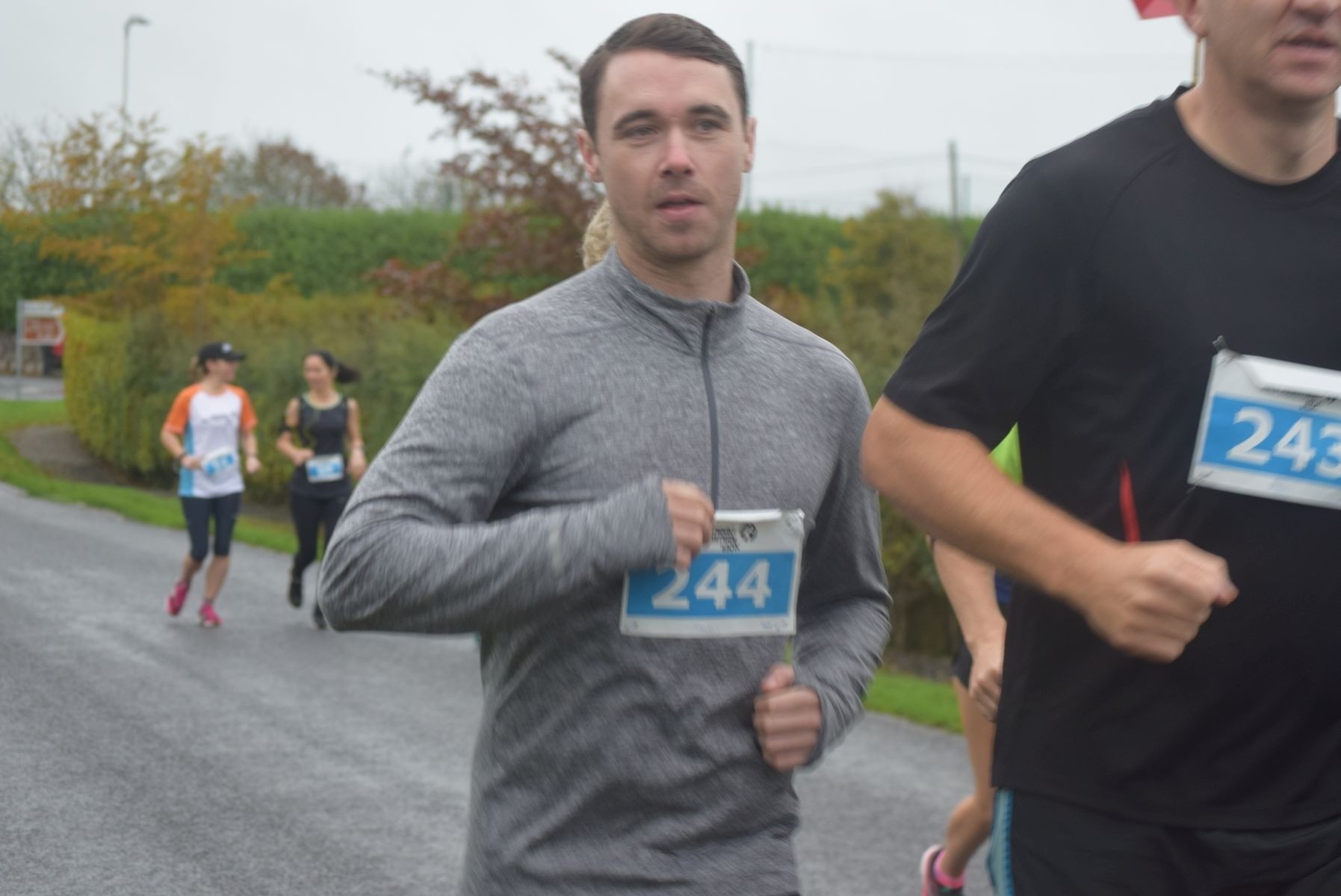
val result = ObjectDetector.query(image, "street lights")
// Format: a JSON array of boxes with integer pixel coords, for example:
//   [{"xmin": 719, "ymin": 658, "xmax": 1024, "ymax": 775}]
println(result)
[{"xmin": 121, "ymin": 16, "xmax": 148, "ymax": 140}]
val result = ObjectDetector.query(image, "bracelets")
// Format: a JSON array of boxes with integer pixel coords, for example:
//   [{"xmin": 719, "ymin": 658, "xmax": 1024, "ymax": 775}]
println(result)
[{"xmin": 177, "ymin": 451, "xmax": 187, "ymax": 460}]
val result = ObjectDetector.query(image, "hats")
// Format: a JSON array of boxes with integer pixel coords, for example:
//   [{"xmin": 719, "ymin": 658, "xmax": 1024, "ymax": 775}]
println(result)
[{"xmin": 196, "ymin": 342, "xmax": 245, "ymax": 366}]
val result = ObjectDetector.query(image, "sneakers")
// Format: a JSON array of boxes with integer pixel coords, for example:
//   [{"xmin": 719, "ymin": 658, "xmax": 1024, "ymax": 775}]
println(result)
[
  {"xmin": 165, "ymin": 579, "xmax": 188, "ymax": 615},
  {"xmin": 921, "ymin": 844, "xmax": 962, "ymax": 896},
  {"xmin": 197, "ymin": 600, "xmax": 220, "ymax": 628}
]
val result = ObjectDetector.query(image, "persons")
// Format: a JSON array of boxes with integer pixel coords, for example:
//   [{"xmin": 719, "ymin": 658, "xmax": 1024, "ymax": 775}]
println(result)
[
  {"xmin": 275, "ymin": 351, "xmax": 368, "ymax": 633},
  {"xmin": 159, "ymin": 341, "xmax": 263, "ymax": 627},
  {"xmin": 917, "ymin": 414, "xmax": 1017, "ymax": 896},
  {"xmin": 856, "ymin": 0, "xmax": 1340, "ymax": 894},
  {"xmin": 319, "ymin": 10, "xmax": 891, "ymax": 895}
]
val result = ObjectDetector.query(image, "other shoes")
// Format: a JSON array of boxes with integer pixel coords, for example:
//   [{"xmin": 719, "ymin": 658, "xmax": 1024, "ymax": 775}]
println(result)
[
  {"xmin": 313, "ymin": 607, "xmax": 325, "ymax": 630},
  {"xmin": 289, "ymin": 573, "xmax": 303, "ymax": 607}
]
